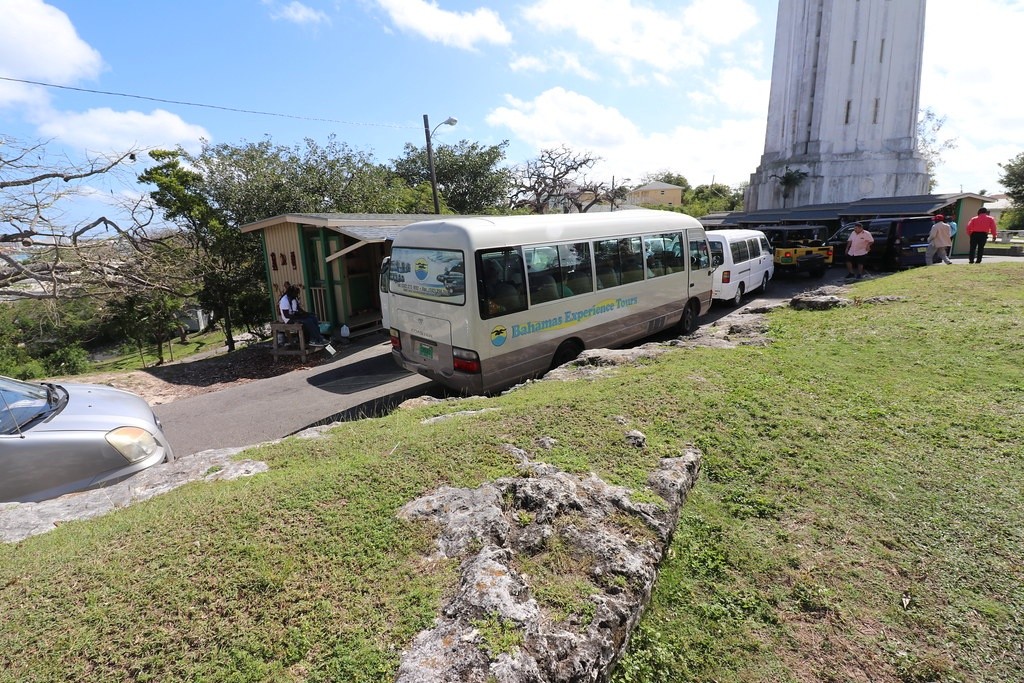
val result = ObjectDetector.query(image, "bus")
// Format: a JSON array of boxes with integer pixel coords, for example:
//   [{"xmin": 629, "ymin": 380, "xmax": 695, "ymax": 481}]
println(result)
[{"xmin": 390, "ymin": 209, "xmax": 713, "ymax": 396}]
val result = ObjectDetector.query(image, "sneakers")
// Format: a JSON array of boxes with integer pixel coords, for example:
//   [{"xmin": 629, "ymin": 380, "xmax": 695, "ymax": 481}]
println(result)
[{"xmin": 309, "ymin": 339, "xmax": 331, "ymax": 346}]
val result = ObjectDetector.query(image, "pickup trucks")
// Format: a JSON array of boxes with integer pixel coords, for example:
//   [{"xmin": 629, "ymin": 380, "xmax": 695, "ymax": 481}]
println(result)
[{"xmin": 759, "ymin": 225, "xmax": 833, "ymax": 279}]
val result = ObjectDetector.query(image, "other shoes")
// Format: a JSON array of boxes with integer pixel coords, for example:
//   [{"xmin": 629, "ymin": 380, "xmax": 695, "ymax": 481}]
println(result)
[
  {"xmin": 845, "ymin": 272, "xmax": 855, "ymax": 278},
  {"xmin": 977, "ymin": 260, "xmax": 980, "ymax": 262},
  {"xmin": 969, "ymin": 259, "xmax": 973, "ymax": 263},
  {"xmin": 857, "ymin": 274, "xmax": 865, "ymax": 278}
]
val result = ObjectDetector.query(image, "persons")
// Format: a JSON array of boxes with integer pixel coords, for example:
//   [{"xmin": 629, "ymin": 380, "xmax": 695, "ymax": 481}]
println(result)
[
  {"xmin": 578, "ymin": 238, "xmax": 673, "ymax": 290},
  {"xmin": 509, "ymin": 272, "xmax": 527, "ymax": 308},
  {"xmin": 844, "ymin": 223, "xmax": 874, "ymax": 279},
  {"xmin": 277, "ymin": 286, "xmax": 332, "ymax": 346},
  {"xmin": 925, "ymin": 214, "xmax": 958, "ymax": 265},
  {"xmin": 552, "ymin": 272, "xmax": 574, "ymax": 297},
  {"xmin": 966, "ymin": 207, "xmax": 997, "ymax": 264}
]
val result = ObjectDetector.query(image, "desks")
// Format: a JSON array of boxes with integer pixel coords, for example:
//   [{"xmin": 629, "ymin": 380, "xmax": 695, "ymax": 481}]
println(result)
[{"xmin": 272, "ymin": 321, "xmax": 324, "ymax": 364}]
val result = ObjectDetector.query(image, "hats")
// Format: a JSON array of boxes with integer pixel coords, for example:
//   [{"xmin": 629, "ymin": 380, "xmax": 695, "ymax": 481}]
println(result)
[{"xmin": 931, "ymin": 214, "xmax": 944, "ymax": 220}]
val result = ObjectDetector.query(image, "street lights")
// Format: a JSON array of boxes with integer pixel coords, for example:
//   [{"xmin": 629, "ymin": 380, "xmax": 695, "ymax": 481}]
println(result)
[{"xmin": 426, "ymin": 117, "xmax": 457, "ymax": 215}]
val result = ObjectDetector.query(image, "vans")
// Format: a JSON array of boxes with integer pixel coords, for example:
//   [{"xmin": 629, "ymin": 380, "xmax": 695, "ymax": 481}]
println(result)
[
  {"xmin": 0, "ymin": 376, "xmax": 174, "ymax": 502},
  {"xmin": 821, "ymin": 216, "xmax": 942, "ymax": 272},
  {"xmin": 704, "ymin": 229, "xmax": 774, "ymax": 305}
]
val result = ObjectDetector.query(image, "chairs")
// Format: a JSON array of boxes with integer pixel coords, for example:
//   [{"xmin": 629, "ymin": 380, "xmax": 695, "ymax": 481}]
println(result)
[{"xmin": 480, "ymin": 251, "xmax": 680, "ymax": 312}]
